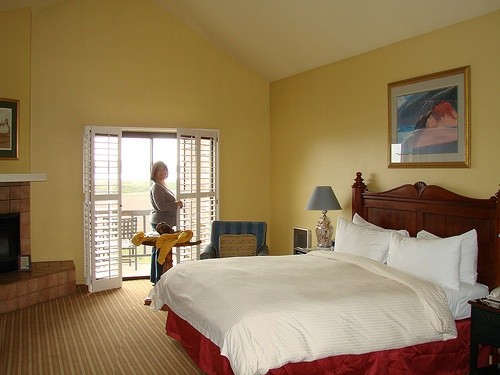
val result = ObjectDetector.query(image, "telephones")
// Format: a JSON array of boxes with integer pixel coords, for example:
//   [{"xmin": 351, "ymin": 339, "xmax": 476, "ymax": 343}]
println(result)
[{"xmin": 488, "ymin": 287, "xmax": 500, "ymax": 301}]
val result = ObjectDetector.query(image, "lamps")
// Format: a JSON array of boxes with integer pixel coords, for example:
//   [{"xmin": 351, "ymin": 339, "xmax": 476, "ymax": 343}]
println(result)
[{"xmin": 306, "ymin": 186, "xmax": 342, "ymax": 248}]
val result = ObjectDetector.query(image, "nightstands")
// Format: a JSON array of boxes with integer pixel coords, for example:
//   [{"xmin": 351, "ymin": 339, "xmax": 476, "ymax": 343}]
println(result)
[
  {"xmin": 467, "ymin": 298, "xmax": 500, "ymax": 375},
  {"xmin": 295, "ymin": 248, "xmax": 332, "ymax": 255}
]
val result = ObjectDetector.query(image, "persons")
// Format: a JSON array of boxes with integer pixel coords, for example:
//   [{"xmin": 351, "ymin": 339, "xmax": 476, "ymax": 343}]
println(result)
[{"xmin": 150, "ymin": 160, "xmax": 183, "ymax": 286}]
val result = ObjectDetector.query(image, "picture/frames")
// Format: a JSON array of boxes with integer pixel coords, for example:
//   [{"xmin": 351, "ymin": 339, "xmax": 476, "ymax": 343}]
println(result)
[
  {"xmin": 0, "ymin": 98, "xmax": 21, "ymax": 161},
  {"xmin": 18, "ymin": 255, "xmax": 32, "ymax": 272},
  {"xmin": 385, "ymin": 63, "xmax": 473, "ymax": 169}
]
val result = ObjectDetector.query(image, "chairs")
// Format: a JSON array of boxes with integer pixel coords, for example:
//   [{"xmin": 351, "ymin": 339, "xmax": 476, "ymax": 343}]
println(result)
[{"xmin": 102, "ymin": 217, "xmax": 138, "ymax": 271}]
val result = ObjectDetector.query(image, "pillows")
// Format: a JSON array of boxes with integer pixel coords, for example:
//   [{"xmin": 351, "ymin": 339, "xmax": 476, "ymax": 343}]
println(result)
[{"xmin": 333, "ymin": 212, "xmax": 479, "ymax": 287}]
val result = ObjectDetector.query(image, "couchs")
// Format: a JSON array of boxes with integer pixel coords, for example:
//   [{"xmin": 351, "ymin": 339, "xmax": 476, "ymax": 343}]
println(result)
[{"xmin": 200, "ymin": 220, "xmax": 270, "ymax": 260}]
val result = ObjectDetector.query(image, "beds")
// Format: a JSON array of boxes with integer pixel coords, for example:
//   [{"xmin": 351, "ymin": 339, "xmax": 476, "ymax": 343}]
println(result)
[{"xmin": 152, "ymin": 172, "xmax": 500, "ymax": 375}]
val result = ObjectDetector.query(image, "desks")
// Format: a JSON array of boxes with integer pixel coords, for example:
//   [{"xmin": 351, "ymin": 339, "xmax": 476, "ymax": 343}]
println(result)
[{"xmin": 141, "ymin": 232, "xmax": 202, "ymax": 305}]
